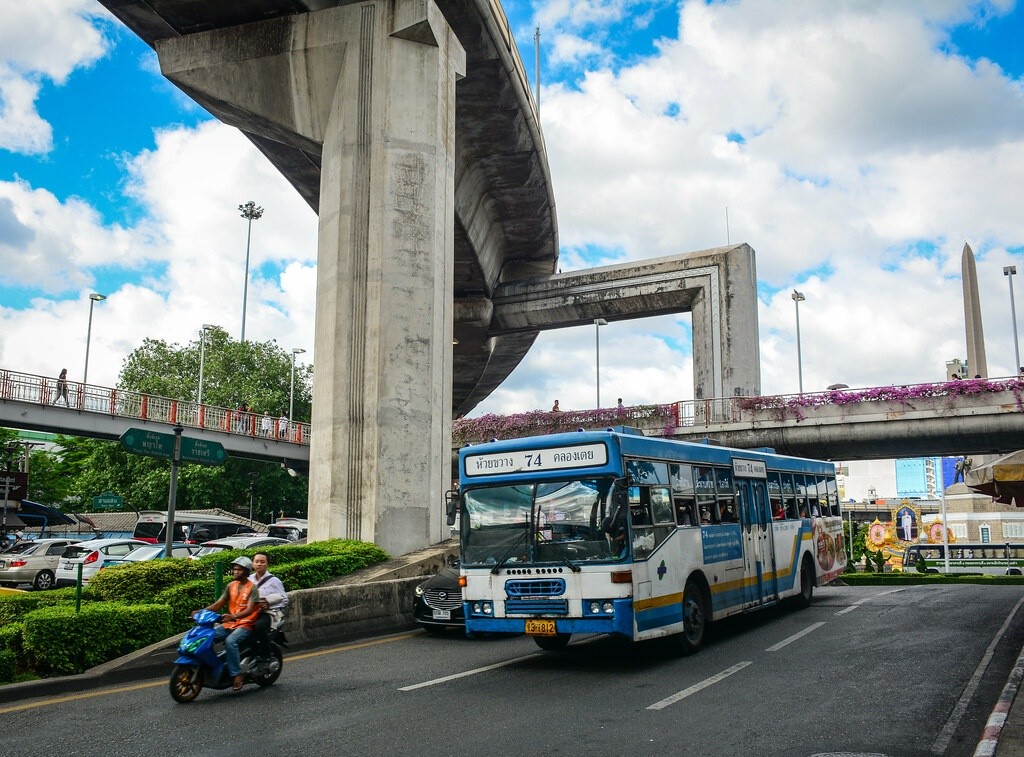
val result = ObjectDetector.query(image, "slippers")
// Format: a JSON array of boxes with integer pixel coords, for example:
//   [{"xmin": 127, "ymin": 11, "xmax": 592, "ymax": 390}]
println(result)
[{"xmin": 231, "ymin": 678, "xmax": 244, "ymax": 691}]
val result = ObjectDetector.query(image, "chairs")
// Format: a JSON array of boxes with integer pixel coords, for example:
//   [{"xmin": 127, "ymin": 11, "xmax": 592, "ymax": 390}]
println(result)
[{"xmin": 678, "ymin": 498, "xmax": 839, "ymax": 526}]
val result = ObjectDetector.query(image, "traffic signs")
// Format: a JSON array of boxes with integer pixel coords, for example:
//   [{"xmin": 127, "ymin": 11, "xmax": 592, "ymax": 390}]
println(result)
[
  {"xmin": 118, "ymin": 426, "xmax": 174, "ymax": 458},
  {"xmin": 178, "ymin": 435, "xmax": 230, "ymax": 468}
]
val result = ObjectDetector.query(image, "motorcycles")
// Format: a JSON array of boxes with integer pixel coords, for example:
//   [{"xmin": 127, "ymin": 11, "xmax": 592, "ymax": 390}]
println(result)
[{"xmin": 169, "ymin": 609, "xmax": 286, "ymax": 704}]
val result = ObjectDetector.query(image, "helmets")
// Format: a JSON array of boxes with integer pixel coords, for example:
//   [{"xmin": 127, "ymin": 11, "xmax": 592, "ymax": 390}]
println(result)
[{"xmin": 231, "ymin": 556, "xmax": 253, "ymax": 570}]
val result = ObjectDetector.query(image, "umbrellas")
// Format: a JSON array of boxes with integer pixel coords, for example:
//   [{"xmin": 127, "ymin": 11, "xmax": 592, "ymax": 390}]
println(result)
[
  {"xmin": 963, "ymin": 448, "xmax": 1023, "ymax": 508},
  {"xmin": 827, "ymin": 383, "xmax": 849, "ymax": 389}
]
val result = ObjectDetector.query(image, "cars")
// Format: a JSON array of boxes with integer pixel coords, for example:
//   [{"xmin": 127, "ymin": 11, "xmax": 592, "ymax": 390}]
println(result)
[
  {"xmin": 55, "ymin": 538, "xmax": 152, "ymax": 587},
  {"xmin": 412, "ymin": 558, "xmax": 466, "ymax": 634},
  {"xmin": 100, "ymin": 510, "xmax": 308, "ymax": 575},
  {"xmin": 0, "ymin": 538, "xmax": 88, "ymax": 591}
]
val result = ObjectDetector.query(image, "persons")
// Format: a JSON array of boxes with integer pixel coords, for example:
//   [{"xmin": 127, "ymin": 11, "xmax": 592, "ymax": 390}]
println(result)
[
  {"xmin": 617, "ymin": 398, "xmax": 625, "ymax": 408},
  {"xmin": 901, "ymin": 510, "xmax": 912, "ymax": 541},
  {"xmin": 279, "ymin": 411, "xmax": 289, "ymax": 439},
  {"xmin": 951, "ymin": 374, "xmax": 962, "ymax": 381},
  {"xmin": 679, "ymin": 511, "xmax": 691, "ymax": 525},
  {"xmin": 15, "ymin": 532, "xmax": 23, "ymax": 539},
  {"xmin": 261, "ymin": 411, "xmax": 272, "ymax": 439},
  {"xmin": 247, "ymin": 552, "xmax": 289, "ymax": 667},
  {"xmin": 786, "ymin": 499, "xmax": 820, "ymax": 518},
  {"xmin": 552, "ymin": 399, "xmax": 560, "ymax": 413},
  {"xmin": 974, "ymin": 375, "xmax": 982, "ymax": 378},
  {"xmin": 718, "ymin": 502, "xmax": 734, "ymax": 522},
  {"xmin": 772, "ymin": 503, "xmax": 785, "ymax": 521},
  {"xmin": 701, "ymin": 511, "xmax": 712, "ymax": 523},
  {"xmin": 604, "ymin": 509, "xmax": 634, "ymax": 541},
  {"xmin": 51, "ymin": 369, "xmax": 70, "ymax": 407},
  {"xmin": 233, "ymin": 402, "xmax": 253, "ymax": 434},
  {"xmin": 188, "ymin": 556, "xmax": 259, "ymax": 690},
  {"xmin": 1019, "ymin": 366, "xmax": 1024, "ymax": 380}
]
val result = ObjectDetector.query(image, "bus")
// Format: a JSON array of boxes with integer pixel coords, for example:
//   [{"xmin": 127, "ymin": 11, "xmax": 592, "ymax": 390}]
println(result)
[
  {"xmin": 445, "ymin": 426, "xmax": 848, "ymax": 655},
  {"xmin": 902, "ymin": 542, "xmax": 1024, "ymax": 575}
]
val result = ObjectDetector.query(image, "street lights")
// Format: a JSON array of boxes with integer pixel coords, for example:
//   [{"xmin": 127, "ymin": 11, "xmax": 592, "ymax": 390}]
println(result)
[
  {"xmin": 198, "ymin": 323, "xmax": 215, "ymax": 428},
  {"xmin": 791, "ymin": 290, "xmax": 807, "ymax": 397},
  {"xmin": 1001, "ymin": 265, "xmax": 1022, "ymax": 381},
  {"xmin": 288, "ymin": 348, "xmax": 306, "ymax": 443},
  {"xmin": 80, "ymin": 294, "xmax": 106, "ymax": 409},
  {"xmin": 591, "ymin": 317, "xmax": 609, "ymax": 411}
]
